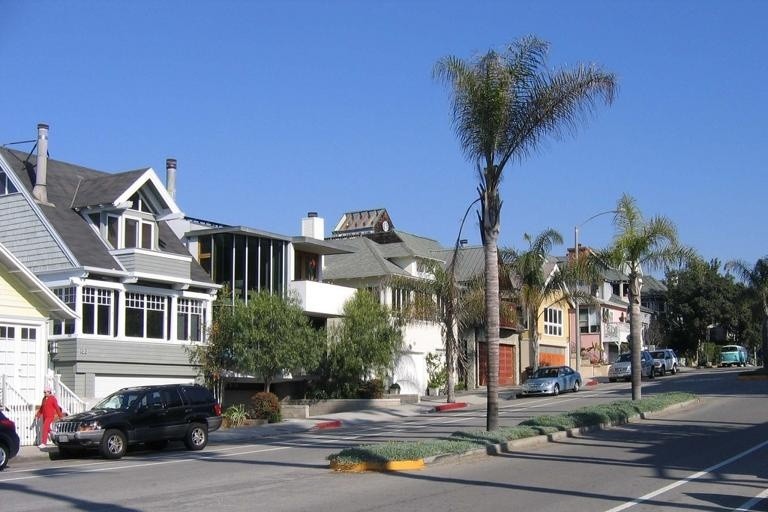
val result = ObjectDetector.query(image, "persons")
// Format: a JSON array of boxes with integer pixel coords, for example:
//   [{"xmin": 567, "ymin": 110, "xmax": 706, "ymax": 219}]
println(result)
[{"xmin": 33, "ymin": 387, "xmax": 65, "ymax": 449}]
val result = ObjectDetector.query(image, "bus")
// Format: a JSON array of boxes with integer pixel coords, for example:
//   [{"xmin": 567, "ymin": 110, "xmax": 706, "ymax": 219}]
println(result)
[{"xmin": 720, "ymin": 344, "xmax": 747, "ymax": 368}]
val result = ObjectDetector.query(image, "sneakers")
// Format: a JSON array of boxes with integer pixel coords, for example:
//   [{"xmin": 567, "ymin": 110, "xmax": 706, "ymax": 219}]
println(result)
[{"xmin": 37, "ymin": 444, "xmax": 47, "ymax": 449}]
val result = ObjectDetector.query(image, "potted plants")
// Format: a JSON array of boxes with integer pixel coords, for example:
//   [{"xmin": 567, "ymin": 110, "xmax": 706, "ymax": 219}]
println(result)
[{"xmin": 424, "ymin": 352, "xmax": 447, "ymax": 397}]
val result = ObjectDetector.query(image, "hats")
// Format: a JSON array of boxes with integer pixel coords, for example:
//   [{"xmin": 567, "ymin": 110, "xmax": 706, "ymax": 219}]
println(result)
[{"xmin": 43, "ymin": 385, "xmax": 51, "ymax": 392}]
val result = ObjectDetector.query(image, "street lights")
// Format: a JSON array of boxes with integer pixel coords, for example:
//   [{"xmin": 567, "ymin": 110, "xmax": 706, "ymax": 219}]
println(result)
[{"xmin": 575, "ymin": 206, "xmax": 627, "ymax": 373}]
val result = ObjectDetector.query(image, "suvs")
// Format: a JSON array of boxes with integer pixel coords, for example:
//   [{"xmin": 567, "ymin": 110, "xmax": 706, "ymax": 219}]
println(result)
[{"xmin": 43, "ymin": 380, "xmax": 225, "ymax": 463}]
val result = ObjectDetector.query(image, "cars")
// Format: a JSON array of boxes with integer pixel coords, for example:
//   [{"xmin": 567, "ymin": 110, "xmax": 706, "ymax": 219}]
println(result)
[
  {"xmin": 605, "ymin": 349, "xmax": 657, "ymax": 383},
  {"xmin": 650, "ymin": 347, "xmax": 680, "ymax": 377},
  {"xmin": 519, "ymin": 364, "xmax": 583, "ymax": 398},
  {"xmin": 0, "ymin": 409, "xmax": 22, "ymax": 472}
]
